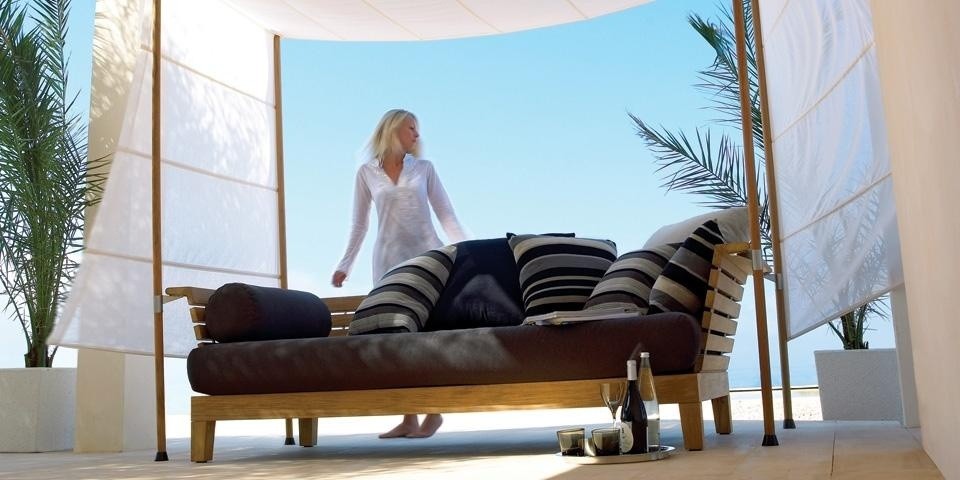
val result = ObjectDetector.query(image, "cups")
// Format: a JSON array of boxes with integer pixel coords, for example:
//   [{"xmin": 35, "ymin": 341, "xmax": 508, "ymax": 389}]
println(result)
[
  {"xmin": 556, "ymin": 428, "xmax": 586, "ymax": 456},
  {"xmin": 591, "ymin": 429, "xmax": 621, "ymax": 456}
]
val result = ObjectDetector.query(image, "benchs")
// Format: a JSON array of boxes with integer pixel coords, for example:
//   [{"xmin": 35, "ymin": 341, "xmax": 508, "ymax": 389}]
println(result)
[{"xmin": 166, "ymin": 242, "xmax": 758, "ymax": 464}]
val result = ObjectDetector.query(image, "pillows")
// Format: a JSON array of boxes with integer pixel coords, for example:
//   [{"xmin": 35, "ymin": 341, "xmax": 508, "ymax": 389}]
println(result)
[
  {"xmin": 582, "ymin": 243, "xmax": 681, "ymax": 315},
  {"xmin": 642, "ymin": 206, "xmax": 754, "ymax": 244},
  {"xmin": 347, "ymin": 244, "xmax": 457, "ymax": 335},
  {"xmin": 648, "ymin": 219, "xmax": 728, "ymax": 315},
  {"xmin": 423, "ymin": 233, "xmax": 575, "ymax": 333},
  {"xmin": 507, "ymin": 233, "xmax": 617, "ymax": 325},
  {"xmin": 204, "ymin": 283, "xmax": 332, "ymax": 341}
]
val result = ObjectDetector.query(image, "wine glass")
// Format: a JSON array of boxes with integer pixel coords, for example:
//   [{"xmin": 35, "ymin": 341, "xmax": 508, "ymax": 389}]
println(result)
[{"xmin": 599, "ymin": 381, "xmax": 626, "ymax": 428}]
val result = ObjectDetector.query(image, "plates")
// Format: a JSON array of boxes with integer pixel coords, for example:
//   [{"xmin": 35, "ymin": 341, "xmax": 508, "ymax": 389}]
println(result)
[{"xmin": 555, "ymin": 444, "xmax": 676, "ymax": 464}]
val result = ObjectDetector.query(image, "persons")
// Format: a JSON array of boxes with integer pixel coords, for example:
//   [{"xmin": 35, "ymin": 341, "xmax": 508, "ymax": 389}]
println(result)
[{"xmin": 332, "ymin": 109, "xmax": 468, "ymax": 438}]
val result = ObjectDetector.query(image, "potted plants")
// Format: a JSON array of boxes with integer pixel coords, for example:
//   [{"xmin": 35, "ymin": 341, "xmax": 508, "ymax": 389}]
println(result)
[
  {"xmin": 0, "ymin": 0, "xmax": 114, "ymax": 453},
  {"xmin": 627, "ymin": 1, "xmax": 901, "ymax": 425}
]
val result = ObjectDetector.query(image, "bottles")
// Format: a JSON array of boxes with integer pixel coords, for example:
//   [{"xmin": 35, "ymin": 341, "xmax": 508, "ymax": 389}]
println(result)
[
  {"xmin": 619, "ymin": 359, "xmax": 650, "ymax": 455},
  {"xmin": 637, "ymin": 350, "xmax": 661, "ymax": 453}
]
